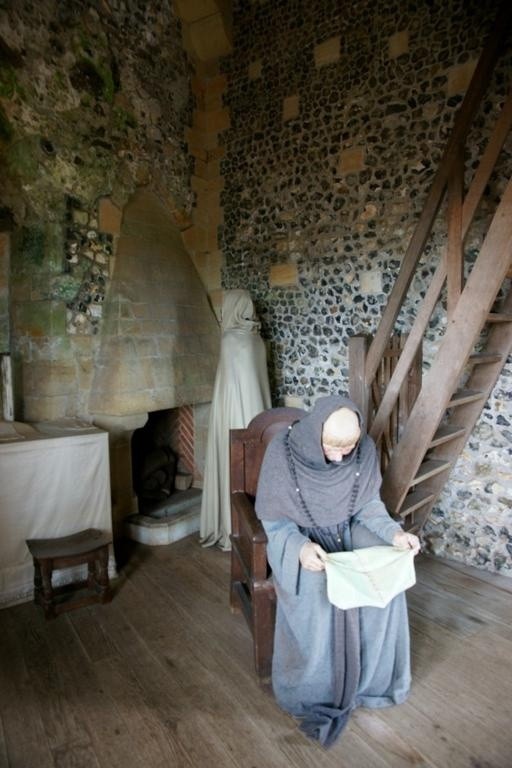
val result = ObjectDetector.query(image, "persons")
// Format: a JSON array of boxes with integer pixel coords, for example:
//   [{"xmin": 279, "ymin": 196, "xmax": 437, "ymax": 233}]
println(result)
[{"xmin": 255, "ymin": 393, "xmax": 422, "ymax": 748}]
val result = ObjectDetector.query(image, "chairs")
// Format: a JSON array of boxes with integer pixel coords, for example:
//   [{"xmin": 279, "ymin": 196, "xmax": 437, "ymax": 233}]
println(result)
[{"xmin": 228, "ymin": 407, "xmax": 405, "ymax": 680}]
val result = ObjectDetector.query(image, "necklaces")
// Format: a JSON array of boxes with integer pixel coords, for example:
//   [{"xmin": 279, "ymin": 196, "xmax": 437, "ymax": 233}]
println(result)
[{"xmin": 284, "ymin": 418, "xmax": 362, "ymax": 543}]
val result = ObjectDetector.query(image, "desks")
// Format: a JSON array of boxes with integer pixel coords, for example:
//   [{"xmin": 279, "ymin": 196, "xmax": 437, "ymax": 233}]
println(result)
[{"xmin": 0, "ymin": 416, "xmax": 120, "ymax": 610}]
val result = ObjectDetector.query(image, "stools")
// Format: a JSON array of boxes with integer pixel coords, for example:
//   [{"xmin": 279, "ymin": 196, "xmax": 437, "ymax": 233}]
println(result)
[{"xmin": 26, "ymin": 527, "xmax": 112, "ymax": 620}]
